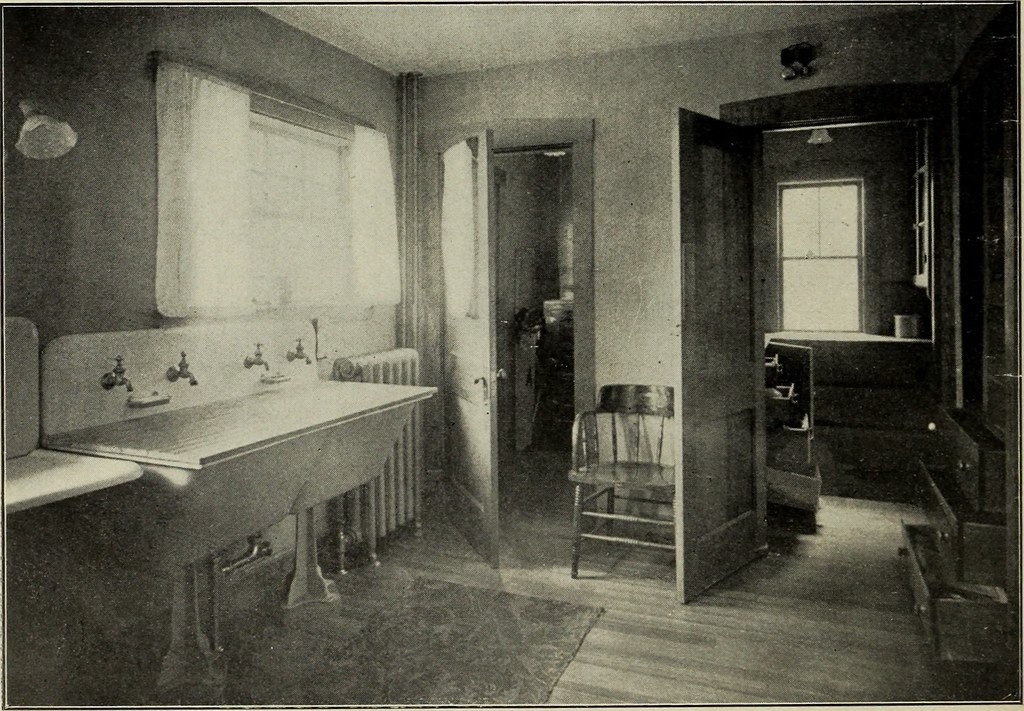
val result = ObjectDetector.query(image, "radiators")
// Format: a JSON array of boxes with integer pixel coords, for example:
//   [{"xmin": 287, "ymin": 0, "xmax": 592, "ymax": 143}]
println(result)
[{"xmin": 328, "ymin": 346, "xmax": 427, "ymax": 572}]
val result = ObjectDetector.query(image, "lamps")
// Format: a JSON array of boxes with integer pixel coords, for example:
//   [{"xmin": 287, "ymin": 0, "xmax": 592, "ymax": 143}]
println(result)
[
  {"xmin": 5, "ymin": 87, "xmax": 81, "ymax": 161},
  {"xmin": 806, "ymin": 127, "xmax": 834, "ymax": 146}
]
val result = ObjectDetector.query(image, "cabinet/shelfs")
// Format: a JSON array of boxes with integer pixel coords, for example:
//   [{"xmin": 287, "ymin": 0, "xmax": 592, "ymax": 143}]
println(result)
[
  {"xmin": 905, "ymin": 116, "xmax": 933, "ymax": 291},
  {"xmin": 892, "ymin": 372, "xmax": 1021, "ymax": 671},
  {"xmin": 762, "ymin": 331, "xmax": 933, "ymax": 513}
]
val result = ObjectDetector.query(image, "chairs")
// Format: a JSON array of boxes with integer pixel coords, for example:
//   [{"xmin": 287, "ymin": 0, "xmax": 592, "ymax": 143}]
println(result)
[{"xmin": 566, "ymin": 383, "xmax": 674, "ymax": 582}]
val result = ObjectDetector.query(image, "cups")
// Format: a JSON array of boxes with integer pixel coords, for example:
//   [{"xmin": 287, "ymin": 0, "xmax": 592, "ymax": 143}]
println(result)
[{"xmin": 894, "ymin": 315, "xmax": 919, "ymax": 338}]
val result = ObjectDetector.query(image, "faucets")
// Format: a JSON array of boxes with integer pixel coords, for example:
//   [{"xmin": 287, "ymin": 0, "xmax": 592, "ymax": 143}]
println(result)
[
  {"xmin": 244, "ymin": 343, "xmax": 270, "ymax": 373},
  {"xmin": 286, "ymin": 339, "xmax": 311, "ymax": 364},
  {"xmin": 103, "ymin": 354, "xmax": 134, "ymax": 391},
  {"xmin": 166, "ymin": 352, "xmax": 198, "ymax": 386}
]
